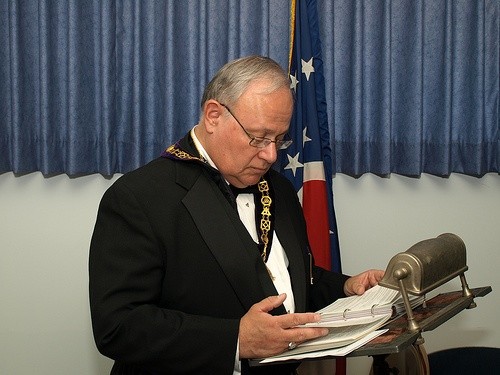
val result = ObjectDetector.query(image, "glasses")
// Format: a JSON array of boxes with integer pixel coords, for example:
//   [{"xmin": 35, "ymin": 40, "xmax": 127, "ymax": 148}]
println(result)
[{"xmin": 220, "ymin": 104, "xmax": 293, "ymax": 150}]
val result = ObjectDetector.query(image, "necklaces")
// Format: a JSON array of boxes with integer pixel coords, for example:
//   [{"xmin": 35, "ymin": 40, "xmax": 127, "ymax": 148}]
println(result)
[{"xmin": 165, "ymin": 145, "xmax": 272, "ymax": 262}]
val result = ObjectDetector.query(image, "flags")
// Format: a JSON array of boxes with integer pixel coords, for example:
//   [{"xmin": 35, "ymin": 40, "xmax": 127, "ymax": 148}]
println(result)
[{"xmin": 278, "ymin": 0, "xmax": 342, "ymax": 273}]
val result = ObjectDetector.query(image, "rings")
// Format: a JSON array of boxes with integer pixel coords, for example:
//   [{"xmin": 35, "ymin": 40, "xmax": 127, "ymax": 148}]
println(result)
[{"xmin": 289, "ymin": 342, "xmax": 297, "ymax": 349}]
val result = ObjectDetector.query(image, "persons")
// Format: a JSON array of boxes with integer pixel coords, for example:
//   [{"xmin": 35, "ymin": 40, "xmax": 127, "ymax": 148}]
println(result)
[{"xmin": 88, "ymin": 55, "xmax": 387, "ymax": 375}]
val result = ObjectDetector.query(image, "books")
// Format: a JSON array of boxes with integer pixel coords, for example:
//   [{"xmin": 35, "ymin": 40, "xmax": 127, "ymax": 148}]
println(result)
[{"xmin": 259, "ymin": 284, "xmax": 426, "ymax": 364}]
